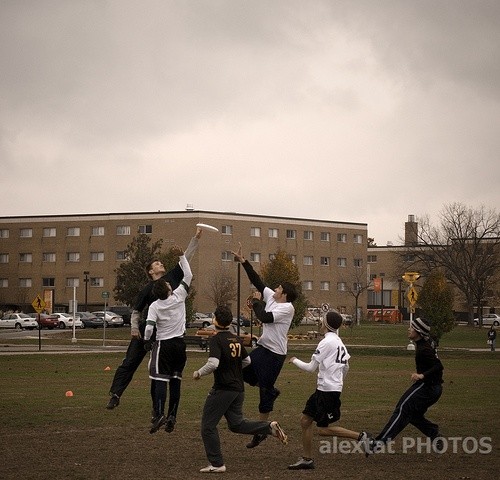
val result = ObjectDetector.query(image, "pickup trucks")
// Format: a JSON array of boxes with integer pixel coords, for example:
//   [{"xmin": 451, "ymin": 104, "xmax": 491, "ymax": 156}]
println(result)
[{"xmin": 300, "ymin": 306, "xmax": 353, "ymax": 325}]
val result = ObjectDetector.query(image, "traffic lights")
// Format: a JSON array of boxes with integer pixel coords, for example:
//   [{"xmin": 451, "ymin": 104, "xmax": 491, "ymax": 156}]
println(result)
[{"xmin": 83, "ymin": 271, "xmax": 89, "ymax": 311}]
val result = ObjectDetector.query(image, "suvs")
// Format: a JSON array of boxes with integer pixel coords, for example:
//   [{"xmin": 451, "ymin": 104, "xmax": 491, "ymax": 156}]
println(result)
[{"xmin": 106, "ymin": 305, "xmax": 132, "ymax": 325}]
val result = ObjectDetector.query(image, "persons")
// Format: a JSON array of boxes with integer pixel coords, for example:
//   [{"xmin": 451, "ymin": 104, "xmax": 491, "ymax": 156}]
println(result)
[
  {"xmin": 366, "ymin": 317, "xmax": 443, "ymax": 459},
  {"xmin": 193, "ymin": 305, "xmax": 289, "ymax": 473},
  {"xmin": 232, "ymin": 241, "xmax": 296, "ymax": 449},
  {"xmin": 488, "ymin": 326, "xmax": 496, "ymax": 351},
  {"xmin": 288, "ymin": 311, "xmax": 372, "ymax": 470},
  {"xmin": 105, "ymin": 223, "xmax": 202, "ymax": 423},
  {"xmin": 144, "ymin": 244, "xmax": 193, "ymax": 434}
]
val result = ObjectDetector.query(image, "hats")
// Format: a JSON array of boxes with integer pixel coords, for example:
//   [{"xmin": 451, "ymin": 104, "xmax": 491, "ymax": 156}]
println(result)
[
  {"xmin": 323, "ymin": 311, "xmax": 342, "ymax": 332},
  {"xmin": 411, "ymin": 318, "xmax": 430, "ymax": 336}
]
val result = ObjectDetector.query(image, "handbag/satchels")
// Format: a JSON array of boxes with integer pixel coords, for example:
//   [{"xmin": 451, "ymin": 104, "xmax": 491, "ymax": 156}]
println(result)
[{"xmin": 487, "ymin": 340, "xmax": 492, "ymax": 344}]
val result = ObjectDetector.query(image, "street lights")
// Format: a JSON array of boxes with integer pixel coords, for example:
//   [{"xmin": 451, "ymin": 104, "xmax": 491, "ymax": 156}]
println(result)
[{"xmin": 233, "ymin": 256, "xmax": 245, "ymax": 336}]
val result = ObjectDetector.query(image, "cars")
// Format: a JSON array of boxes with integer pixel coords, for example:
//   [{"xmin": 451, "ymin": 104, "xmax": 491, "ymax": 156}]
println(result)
[
  {"xmin": 195, "ymin": 324, "xmax": 260, "ymax": 348},
  {"xmin": 188, "ymin": 313, "xmax": 212, "ymax": 328},
  {"xmin": 28, "ymin": 312, "xmax": 59, "ymax": 329},
  {"xmin": 205, "ymin": 312, "xmax": 244, "ymax": 325},
  {"xmin": 50, "ymin": 313, "xmax": 82, "ymax": 329},
  {"xmin": 474, "ymin": 313, "xmax": 500, "ymax": 326},
  {"xmin": 0, "ymin": 313, "xmax": 38, "ymax": 331},
  {"xmin": 92, "ymin": 311, "xmax": 124, "ymax": 328},
  {"xmin": 72, "ymin": 311, "xmax": 103, "ymax": 328}
]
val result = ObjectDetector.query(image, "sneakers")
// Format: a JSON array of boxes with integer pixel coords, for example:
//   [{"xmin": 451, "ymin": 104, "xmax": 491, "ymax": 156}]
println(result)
[
  {"xmin": 434, "ymin": 434, "xmax": 444, "ymax": 450},
  {"xmin": 200, "ymin": 465, "xmax": 226, "ymax": 472},
  {"xmin": 362, "ymin": 432, "xmax": 373, "ymax": 457},
  {"xmin": 150, "ymin": 415, "xmax": 166, "ymax": 434},
  {"xmin": 270, "ymin": 422, "xmax": 288, "ymax": 446},
  {"xmin": 152, "ymin": 416, "xmax": 176, "ymax": 424},
  {"xmin": 289, "ymin": 457, "xmax": 314, "ymax": 470},
  {"xmin": 107, "ymin": 397, "xmax": 119, "ymax": 409},
  {"xmin": 246, "ymin": 434, "xmax": 267, "ymax": 448},
  {"xmin": 165, "ymin": 416, "xmax": 175, "ymax": 433}
]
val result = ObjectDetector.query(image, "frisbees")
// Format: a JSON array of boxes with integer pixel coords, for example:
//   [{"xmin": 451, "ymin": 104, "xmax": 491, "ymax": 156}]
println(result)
[{"xmin": 196, "ymin": 224, "xmax": 219, "ymax": 232}]
